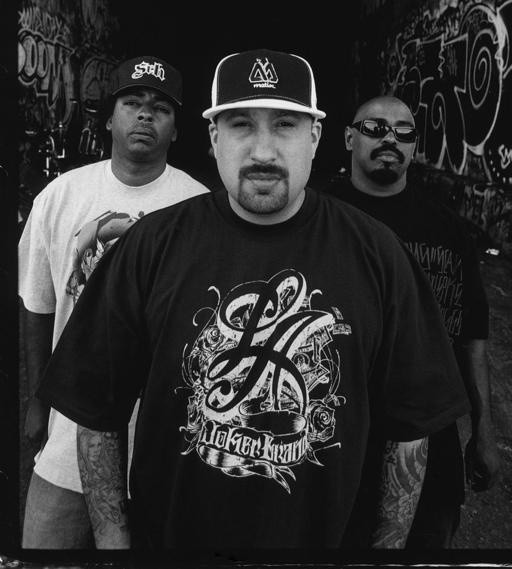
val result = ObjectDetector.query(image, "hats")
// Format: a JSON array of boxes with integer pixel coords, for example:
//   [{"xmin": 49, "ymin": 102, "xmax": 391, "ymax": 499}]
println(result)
[
  {"xmin": 201, "ymin": 50, "xmax": 327, "ymax": 121},
  {"xmin": 109, "ymin": 55, "xmax": 188, "ymax": 107}
]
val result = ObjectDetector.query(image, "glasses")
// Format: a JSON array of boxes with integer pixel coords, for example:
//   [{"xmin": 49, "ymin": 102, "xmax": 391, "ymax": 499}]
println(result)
[{"xmin": 352, "ymin": 119, "xmax": 418, "ymax": 144}]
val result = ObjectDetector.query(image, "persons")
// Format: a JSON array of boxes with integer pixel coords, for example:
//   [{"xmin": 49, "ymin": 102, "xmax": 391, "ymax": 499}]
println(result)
[
  {"xmin": 306, "ymin": 95, "xmax": 500, "ymax": 549},
  {"xmin": 34, "ymin": 50, "xmax": 471, "ymax": 551},
  {"xmin": 13, "ymin": 48, "xmax": 208, "ymax": 549}
]
